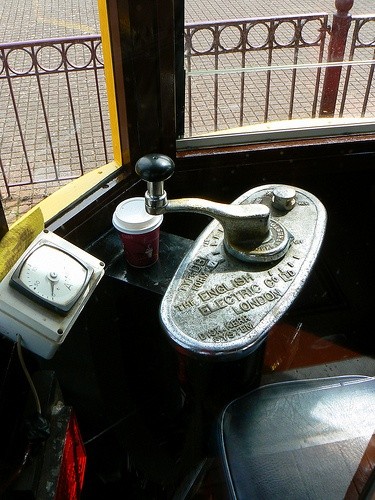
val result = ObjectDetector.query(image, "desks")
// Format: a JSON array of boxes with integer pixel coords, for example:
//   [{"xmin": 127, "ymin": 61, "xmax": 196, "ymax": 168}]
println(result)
[{"xmin": 85, "ymin": 225, "xmax": 195, "ymax": 383}]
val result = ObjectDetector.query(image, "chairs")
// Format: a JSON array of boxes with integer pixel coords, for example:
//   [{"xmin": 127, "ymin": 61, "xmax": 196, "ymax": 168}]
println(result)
[{"xmin": 215, "ymin": 373, "xmax": 374, "ymax": 500}]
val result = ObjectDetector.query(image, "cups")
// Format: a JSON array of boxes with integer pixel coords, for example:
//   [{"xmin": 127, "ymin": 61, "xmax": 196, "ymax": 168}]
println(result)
[{"xmin": 113, "ymin": 198, "xmax": 163, "ymax": 268}]
